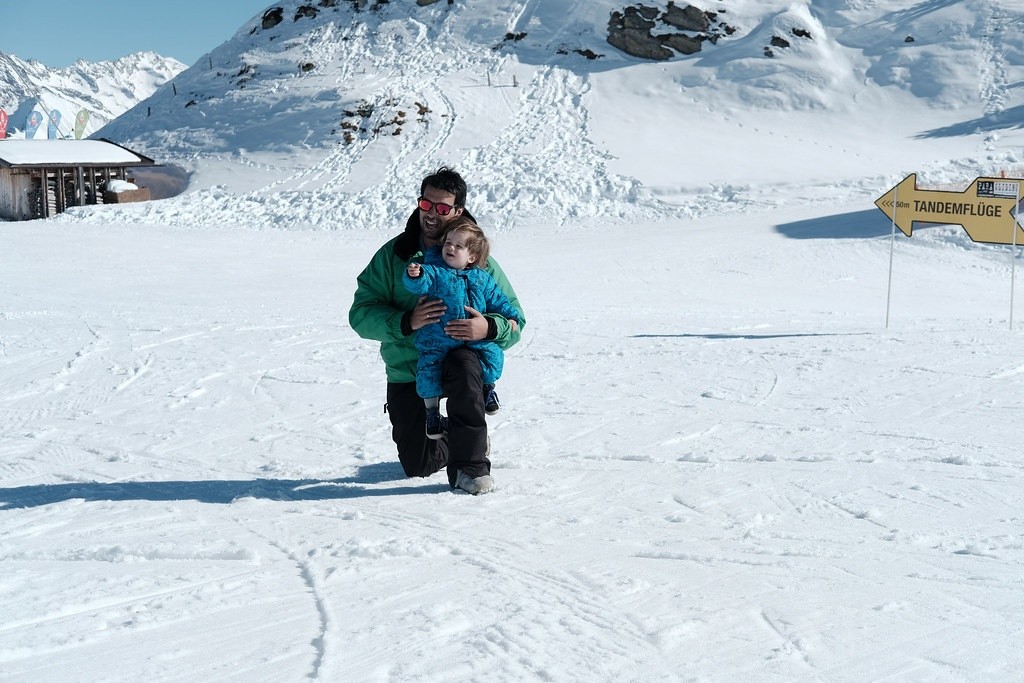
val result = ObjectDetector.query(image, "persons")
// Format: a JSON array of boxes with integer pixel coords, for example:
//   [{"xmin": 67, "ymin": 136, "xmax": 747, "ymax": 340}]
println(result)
[
  {"xmin": 349, "ymin": 164, "xmax": 522, "ymax": 498},
  {"xmin": 402, "ymin": 215, "xmax": 519, "ymax": 441}
]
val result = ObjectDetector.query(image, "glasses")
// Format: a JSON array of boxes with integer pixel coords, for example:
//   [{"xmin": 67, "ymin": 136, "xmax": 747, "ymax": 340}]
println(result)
[{"xmin": 417, "ymin": 196, "xmax": 459, "ymax": 216}]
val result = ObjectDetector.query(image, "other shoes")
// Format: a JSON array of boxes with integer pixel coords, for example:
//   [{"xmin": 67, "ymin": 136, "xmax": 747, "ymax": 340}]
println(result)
[
  {"xmin": 486, "ymin": 435, "xmax": 491, "ymax": 456},
  {"xmin": 454, "ymin": 469, "xmax": 495, "ymax": 495}
]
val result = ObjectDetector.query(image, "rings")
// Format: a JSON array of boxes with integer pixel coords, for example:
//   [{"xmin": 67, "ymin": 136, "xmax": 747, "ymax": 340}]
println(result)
[{"xmin": 426, "ymin": 313, "xmax": 430, "ymax": 319}]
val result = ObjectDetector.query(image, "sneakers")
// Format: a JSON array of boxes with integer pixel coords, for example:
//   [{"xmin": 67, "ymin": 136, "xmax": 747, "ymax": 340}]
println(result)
[
  {"xmin": 484, "ymin": 390, "xmax": 501, "ymax": 415},
  {"xmin": 425, "ymin": 402, "xmax": 445, "ymax": 439}
]
